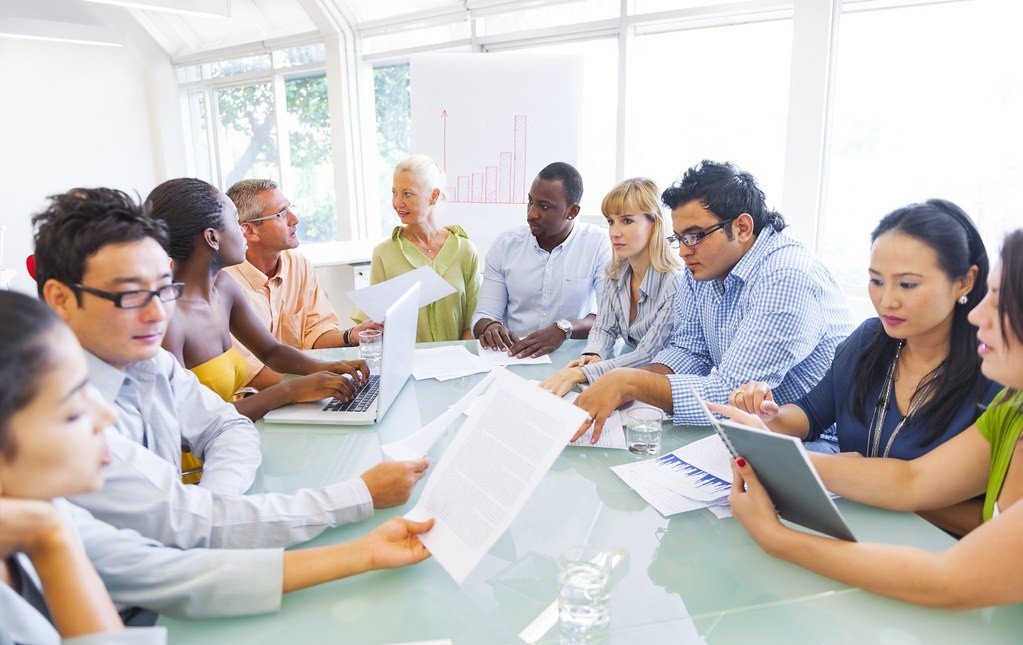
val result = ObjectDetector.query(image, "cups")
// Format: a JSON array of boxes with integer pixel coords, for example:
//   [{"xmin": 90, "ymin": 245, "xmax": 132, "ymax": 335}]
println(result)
[
  {"xmin": 627, "ymin": 407, "xmax": 664, "ymax": 459},
  {"xmin": 358, "ymin": 330, "xmax": 382, "ymax": 362},
  {"xmin": 557, "ymin": 544, "xmax": 610, "ymax": 644}
]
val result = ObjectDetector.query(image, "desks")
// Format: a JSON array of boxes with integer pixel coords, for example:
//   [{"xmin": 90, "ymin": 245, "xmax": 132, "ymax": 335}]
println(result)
[
  {"xmin": 154, "ymin": 339, "xmax": 1023, "ymax": 645},
  {"xmin": 293, "ymin": 241, "xmax": 376, "ymax": 297}
]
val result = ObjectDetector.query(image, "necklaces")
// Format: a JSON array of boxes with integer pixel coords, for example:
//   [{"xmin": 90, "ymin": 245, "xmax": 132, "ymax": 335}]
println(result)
[{"xmin": 405, "ymin": 233, "xmax": 438, "ymax": 253}]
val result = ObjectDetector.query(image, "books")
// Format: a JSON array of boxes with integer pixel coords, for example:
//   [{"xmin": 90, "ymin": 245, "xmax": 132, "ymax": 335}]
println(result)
[{"xmin": 688, "ymin": 386, "xmax": 857, "ymax": 543}]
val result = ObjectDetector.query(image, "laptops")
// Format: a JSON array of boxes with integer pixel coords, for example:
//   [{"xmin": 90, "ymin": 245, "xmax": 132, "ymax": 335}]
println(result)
[{"xmin": 264, "ymin": 281, "xmax": 422, "ymax": 425}]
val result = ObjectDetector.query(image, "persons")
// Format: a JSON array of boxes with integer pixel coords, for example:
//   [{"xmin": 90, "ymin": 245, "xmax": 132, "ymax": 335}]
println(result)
[
  {"xmin": 470, "ymin": 162, "xmax": 617, "ymax": 360},
  {"xmin": 704, "ymin": 227, "xmax": 1023, "ymax": 610},
  {"xmin": 144, "ymin": 179, "xmax": 370, "ymax": 483},
  {"xmin": 538, "ymin": 178, "xmax": 683, "ymax": 397},
  {"xmin": 0, "ymin": 288, "xmax": 436, "ymax": 645},
  {"xmin": 348, "ymin": 155, "xmax": 480, "ymax": 344},
  {"xmin": 728, "ymin": 198, "xmax": 1008, "ymax": 539},
  {"xmin": 32, "ymin": 186, "xmax": 431, "ymax": 627},
  {"xmin": 570, "ymin": 160, "xmax": 856, "ymax": 444},
  {"xmin": 222, "ymin": 180, "xmax": 384, "ymax": 350}
]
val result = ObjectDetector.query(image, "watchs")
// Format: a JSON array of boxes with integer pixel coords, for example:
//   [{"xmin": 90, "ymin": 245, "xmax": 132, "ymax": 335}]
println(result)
[{"xmin": 555, "ymin": 319, "xmax": 572, "ymax": 340}]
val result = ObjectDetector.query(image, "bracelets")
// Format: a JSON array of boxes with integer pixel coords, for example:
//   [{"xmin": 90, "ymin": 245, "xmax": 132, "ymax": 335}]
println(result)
[
  {"xmin": 482, "ymin": 321, "xmax": 502, "ymax": 333},
  {"xmin": 343, "ymin": 327, "xmax": 355, "ymax": 346}
]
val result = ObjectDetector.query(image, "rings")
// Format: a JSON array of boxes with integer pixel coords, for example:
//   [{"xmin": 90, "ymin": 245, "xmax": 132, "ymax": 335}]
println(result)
[
  {"xmin": 735, "ymin": 391, "xmax": 743, "ymax": 396},
  {"xmin": 588, "ymin": 416, "xmax": 594, "ymax": 422}
]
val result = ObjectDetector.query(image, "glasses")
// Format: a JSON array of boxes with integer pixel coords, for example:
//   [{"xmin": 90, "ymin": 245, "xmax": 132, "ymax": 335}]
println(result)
[
  {"xmin": 666, "ymin": 217, "xmax": 736, "ymax": 248},
  {"xmin": 246, "ymin": 203, "xmax": 294, "ymax": 224},
  {"xmin": 72, "ymin": 279, "xmax": 186, "ymax": 309}
]
